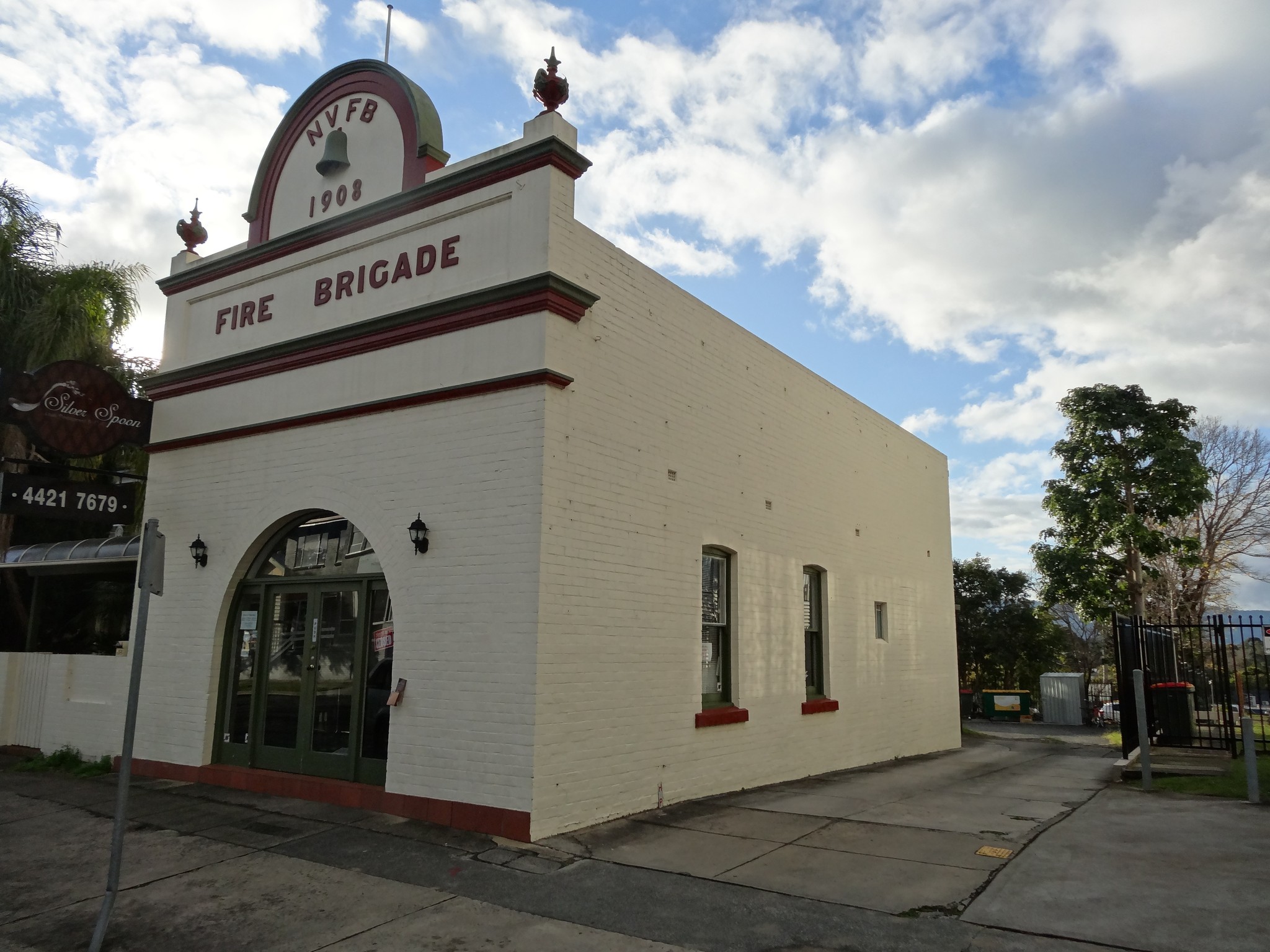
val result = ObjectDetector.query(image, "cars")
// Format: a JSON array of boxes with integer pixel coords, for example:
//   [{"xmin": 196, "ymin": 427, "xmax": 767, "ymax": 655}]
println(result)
[{"xmin": 1096, "ymin": 702, "xmax": 1123, "ymax": 724}]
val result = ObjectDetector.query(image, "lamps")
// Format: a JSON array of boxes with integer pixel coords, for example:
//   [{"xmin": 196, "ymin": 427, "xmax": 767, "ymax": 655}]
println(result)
[
  {"xmin": 406, "ymin": 511, "xmax": 431, "ymax": 556},
  {"xmin": 187, "ymin": 533, "xmax": 208, "ymax": 569}
]
